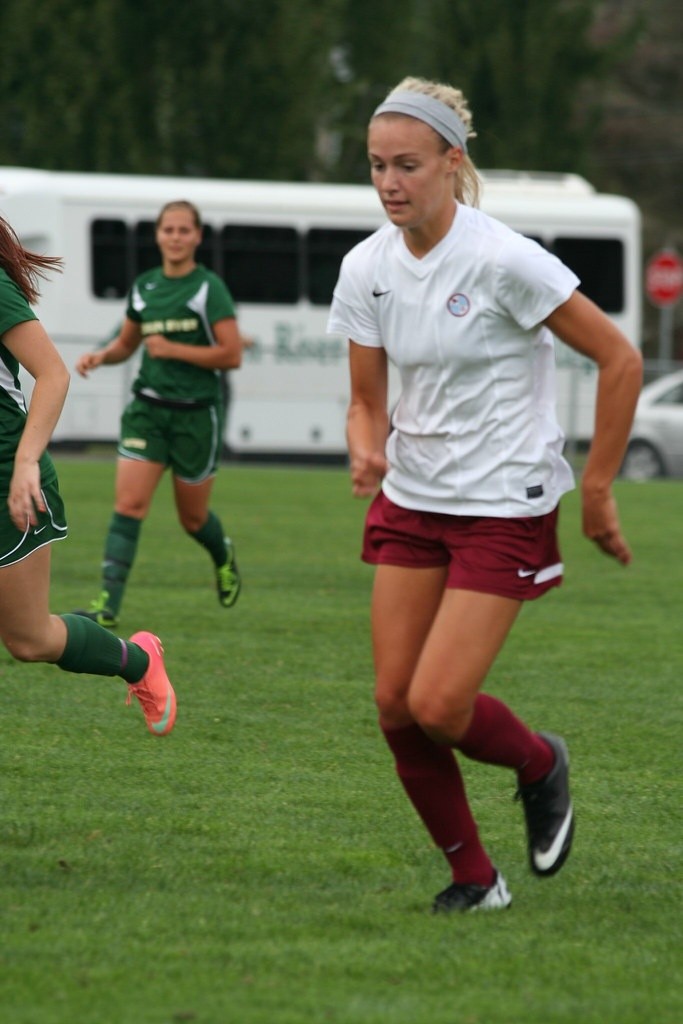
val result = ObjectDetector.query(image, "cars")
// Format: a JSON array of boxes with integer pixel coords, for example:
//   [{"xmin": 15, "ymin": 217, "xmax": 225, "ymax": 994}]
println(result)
[{"xmin": 616, "ymin": 373, "xmax": 683, "ymax": 484}]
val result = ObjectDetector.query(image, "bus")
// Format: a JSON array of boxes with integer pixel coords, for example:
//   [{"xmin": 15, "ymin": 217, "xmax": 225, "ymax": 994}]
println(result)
[{"xmin": 0, "ymin": 162, "xmax": 645, "ymax": 459}]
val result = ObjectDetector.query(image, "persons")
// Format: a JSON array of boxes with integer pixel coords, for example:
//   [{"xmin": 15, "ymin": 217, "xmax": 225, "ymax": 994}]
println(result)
[
  {"xmin": 326, "ymin": 74, "xmax": 644, "ymax": 919},
  {"xmin": 70, "ymin": 200, "xmax": 245, "ymax": 628},
  {"xmin": 0, "ymin": 214, "xmax": 177, "ymax": 739}
]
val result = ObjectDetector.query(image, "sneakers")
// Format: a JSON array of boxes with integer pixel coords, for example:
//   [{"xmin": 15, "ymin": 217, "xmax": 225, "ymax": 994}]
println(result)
[
  {"xmin": 509, "ymin": 732, "xmax": 575, "ymax": 880},
  {"xmin": 431, "ymin": 868, "xmax": 513, "ymax": 920},
  {"xmin": 214, "ymin": 537, "xmax": 240, "ymax": 609},
  {"xmin": 126, "ymin": 630, "xmax": 177, "ymax": 737},
  {"xmin": 73, "ymin": 591, "xmax": 118, "ymax": 632}
]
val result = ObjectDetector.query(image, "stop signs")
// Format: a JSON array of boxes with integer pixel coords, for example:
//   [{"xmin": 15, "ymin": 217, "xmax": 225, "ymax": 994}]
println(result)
[{"xmin": 647, "ymin": 255, "xmax": 683, "ymax": 306}]
